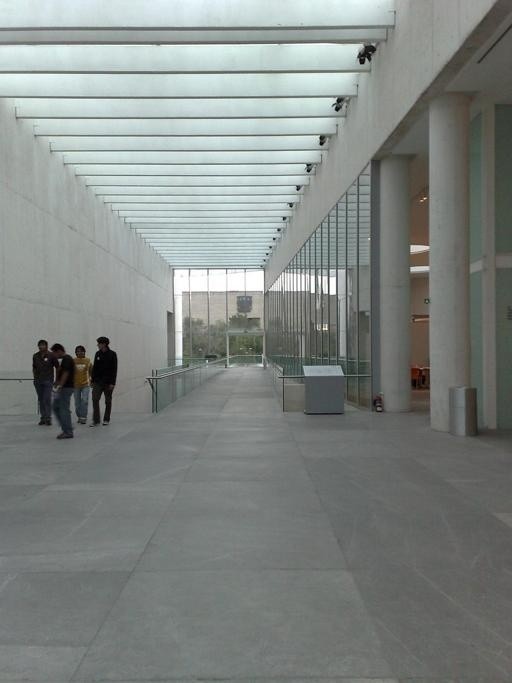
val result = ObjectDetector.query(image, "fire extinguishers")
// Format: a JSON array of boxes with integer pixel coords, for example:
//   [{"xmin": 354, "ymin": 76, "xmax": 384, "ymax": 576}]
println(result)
[{"xmin": 373, "ymin": 392, "xmax": 383, "ymax": 412}]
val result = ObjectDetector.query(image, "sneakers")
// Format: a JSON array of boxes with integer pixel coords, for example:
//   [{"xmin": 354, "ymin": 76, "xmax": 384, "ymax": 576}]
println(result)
[
  {"xmin": 103, "ymin": 420, "xmax": 109, "ymax": 425},
  {"xmin": 77, "ymin": 418, "xmax": 86, "ymax": 424},
  {"xmin": 38, "ymin": 418, "xmax": 52, "ymax": 426},
  {"xmin": 90, "ymin": 421, "xmax": 100, "ymax": 427},
  {"xmin": 57, "ymin": 432, "xmax": 73, "ymax": 439}
]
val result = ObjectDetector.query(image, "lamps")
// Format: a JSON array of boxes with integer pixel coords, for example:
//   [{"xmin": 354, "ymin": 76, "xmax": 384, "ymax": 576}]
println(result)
[{"xmin": 260, "ymin": 43, "xmax": 377, "ymax": 269}]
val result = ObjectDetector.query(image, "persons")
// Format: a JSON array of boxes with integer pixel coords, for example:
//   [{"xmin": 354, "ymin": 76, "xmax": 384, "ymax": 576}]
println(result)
[
  {"xmin": 50, "ymin": 342, "xmax": 75, "ymax": 439},
  {"xmin": 88, "ymin": 335, "xmax": 118, "ymax": 427},
  {"xmin": 32, "ymin": 338, "xmax": 60, "ymax": 426},
  {"xmin": 72, "ymin": 344, "xmax": 95, "ymax": 425}
]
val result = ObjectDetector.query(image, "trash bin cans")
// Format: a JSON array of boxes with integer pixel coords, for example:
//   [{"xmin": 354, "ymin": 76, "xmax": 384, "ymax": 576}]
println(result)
[{"xmin": 448, "ymin": 384, "xmax": 477, "ymax": 437}]
